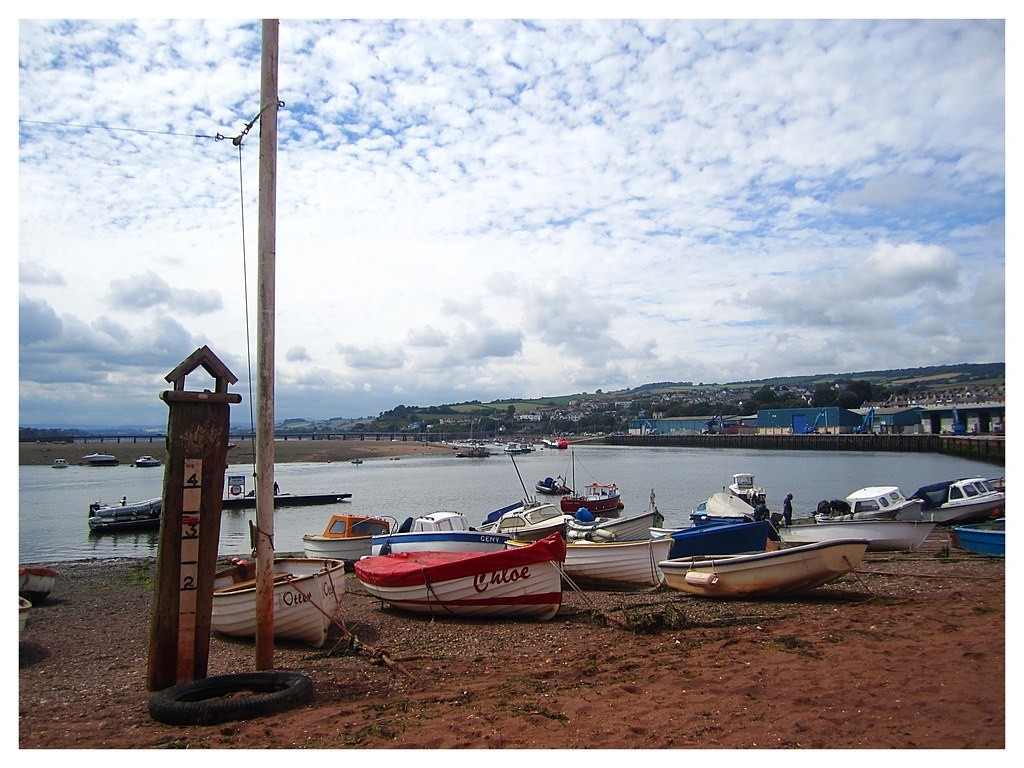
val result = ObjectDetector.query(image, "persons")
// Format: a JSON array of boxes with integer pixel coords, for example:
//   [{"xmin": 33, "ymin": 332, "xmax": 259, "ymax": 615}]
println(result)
[
  {"xmin": 782, "ymin": 492, "xmax": 794, "ymax": 526},
  {"xmin": 817, "ymin": 499, "xmax": 852, "ymax": 519},
  {"xmin": 274, "ymin": 481, "xmax": 280, "ymax": 496}
]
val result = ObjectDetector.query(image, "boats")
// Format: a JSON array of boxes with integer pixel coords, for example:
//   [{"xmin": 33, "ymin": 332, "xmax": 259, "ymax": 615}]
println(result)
[
  {"xmin": 51, "ymin": 459, "xmax": 69, "ymax": 467},
  {"xmin": 87, "ymin": 496, "xmax": 161, "ymax": 532},
  {"xmin": 370, "ymin": 517, "xmax": 512, "ymax": 556},
  {"xmin": 398, "ymin": 454, "xmax": 770, "ymax": 596},
  {"xmin": 19, "ymin": 565, "xmax": 60, "ymax": 638},
  {"xmin": 729, "ymin": 473, "xmax": 766, "ymax": 506},
  {"xmin": 907, "ymin": 477, "xmax": 1003, "ymax": 523},
  {"xmin": 301, "ymin": 513, "xmax": 394, "ymax": 569},
  {"xmin": 954, "ymin": 520, "xmax": 1005, "ymax": 558},
  {"xmin": 536, "ymin": 477, "xmax": 571, "ymax": 495},
  {"xmin": 90, "ymin": 460, "xmax": 119, "ymax": 466},
  {"xmin": 453, "ymin": 438, "xmax": 568, "ymax": 457},
  {"xmin": 353, "ymin": 531, "xmax": 567, "ymax": 621},
  {"xmin": 560, "ymin": 448, "xmax": 620, "ymax": 514},
  {"xmin": 211, "ymin": 557, "xmax": 346, "ymax": 649},
  {"xmin": 658, "ymin": 537, "xmax": 869, "ymax": 597},
  {"xmin": 814, "ymin": 486, "xmax": 924, "ymax": 522},
  {"xmin": 769, "ymin": 513, "xmax": 937, "ymax": 551},
  {"xmin": 83, "ymin": 452, "xmax": 115, "ymax": 461},
  {"xmin": 135, "ymin": 456, "xmax": 160, "ymax": 467}
]
